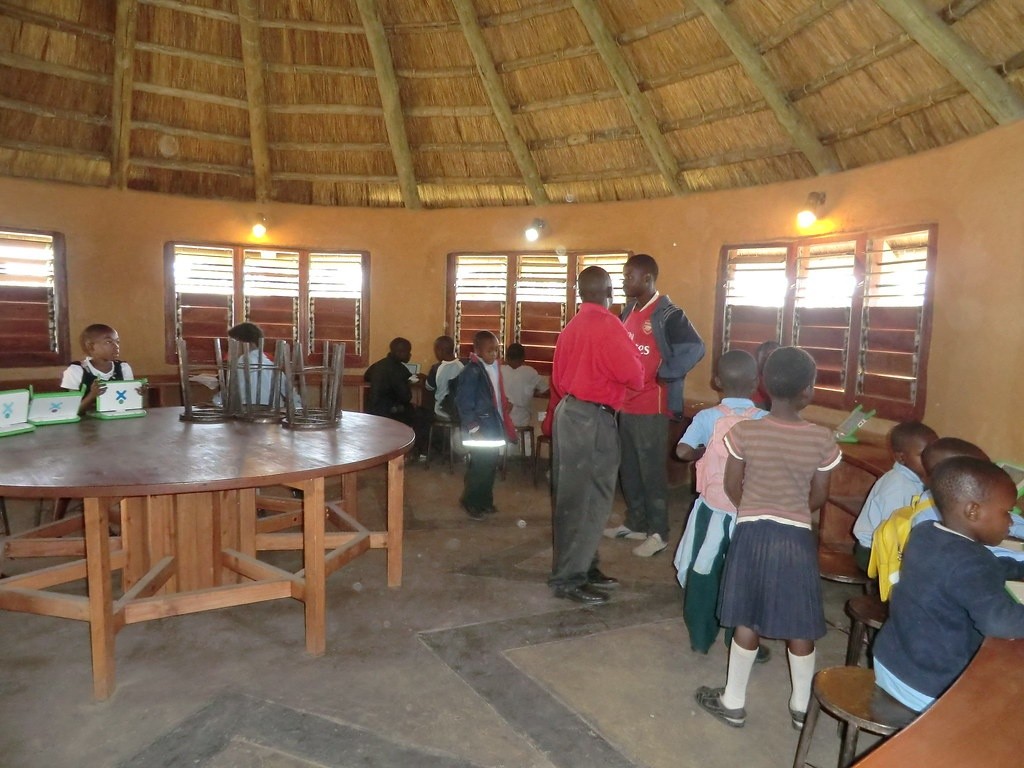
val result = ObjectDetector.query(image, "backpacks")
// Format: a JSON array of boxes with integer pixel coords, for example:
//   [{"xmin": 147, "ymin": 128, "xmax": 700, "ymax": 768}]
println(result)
[
  {"xmin": 439, "ymin": 374, "xmax": 462, "ymax": 427},
  {"xmin": 693, "ymin": 406, "xmax": 763, "ymax": 511},
  {"xmin": 867, "ymin": 495, "xmax": 936, "ymax": 602}
]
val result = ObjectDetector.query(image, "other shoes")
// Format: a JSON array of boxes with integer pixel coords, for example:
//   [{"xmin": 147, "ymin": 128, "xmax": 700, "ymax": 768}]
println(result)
[
  {"xmin": 482, "ymin": 501, "xmax": 495, "ymax": 513},
  {"xmin": 751, "ymin": 644, "xmax": 770, "ymax": 663},
  {"xmin": 689, "ymin": 639, "xmax": 713, "ymax": 655},
  {"xmin": 462, "ymin": 505, "xmax": 486, "ymax": 523}
]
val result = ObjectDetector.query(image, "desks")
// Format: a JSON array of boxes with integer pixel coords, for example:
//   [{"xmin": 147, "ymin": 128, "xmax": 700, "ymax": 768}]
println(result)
[{"xmin": 0, "ymin": 404, "xmax": 416, "ymax": 702}]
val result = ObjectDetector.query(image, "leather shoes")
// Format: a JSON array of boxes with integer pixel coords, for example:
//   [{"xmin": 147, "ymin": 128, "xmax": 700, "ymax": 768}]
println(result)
[
  {"xmin": 554, "ymin": 584, "xmax": 608, "ymax": 605},
  {"xmin": 588, "ymin": 566, "xmax": 618, "ymax": 586}
]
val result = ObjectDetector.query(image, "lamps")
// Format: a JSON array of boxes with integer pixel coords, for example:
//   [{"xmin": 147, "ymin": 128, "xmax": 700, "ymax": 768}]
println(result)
[
  {"xmin": 253, "ymin": 211, "xmax": 268, "ymax": 238},
  {"xmin": 799, "ymin": 189, "xmax": 826, "ymax": 228},
  {"xmin": 526, "ymin": 218, "xmax": 545, "ymax": 240}
]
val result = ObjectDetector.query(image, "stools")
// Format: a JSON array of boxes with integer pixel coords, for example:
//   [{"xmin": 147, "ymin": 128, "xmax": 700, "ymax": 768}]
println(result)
[
  {"xmin": 425, "ymin": 411, "xmax": 924, "ymax": 768},
  {"xmin": 174, "ymin": 335, "xmax": 345, "ymax": 430}
]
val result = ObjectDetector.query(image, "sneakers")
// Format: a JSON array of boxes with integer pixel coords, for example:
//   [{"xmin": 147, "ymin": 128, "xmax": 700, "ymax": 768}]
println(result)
[
  {"xmin": 786, "ymin": 698, "xmax": 807, "ymax": 731},
  {"xmin": 633, "ymin": 533, "xmax": 669, "ymax": 558},
  {"xmin": 601, "ymin": 525, "xmax": 647, "ymax": 541},
  {"xmin": 694, "ymin": 685, "xmax": 745, "ymax": 727}
]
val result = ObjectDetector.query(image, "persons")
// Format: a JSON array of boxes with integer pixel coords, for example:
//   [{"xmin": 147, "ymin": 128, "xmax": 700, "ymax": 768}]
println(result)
[
  {"xmin": 362, "ymin": 337, "xmax": 431, "ymax": 462},
  {"xmin": 416, "ymin": 336, "xmax": 469, "ymax": 461},
  {"xmin": 674, "ymin": 340, "xmax": 843, "ymax": 731},
  {"xmin": 58, "ymin": 324, "xmax": 149, "ymax": 416},
  {"xmin": 452, "ymin": 330, "xmax": 519, "ymax": 520},
  {"xmin": 546, "ymin": 266, "xmax": 645, "ymax": 605},
  {"xmin": 210, "ymin": 322, "xmax": 302, "ymax": 409},
  {"xmin": 851, "ymin": 420, "xmax": 1024, "ymax": 715},
  {"xmin": 497, "ymin": 342, "xmax": 550, "ymax": 467},
  {"xmin": 603, "ymin": 254, "xmax": 704, "ymax": 558}
]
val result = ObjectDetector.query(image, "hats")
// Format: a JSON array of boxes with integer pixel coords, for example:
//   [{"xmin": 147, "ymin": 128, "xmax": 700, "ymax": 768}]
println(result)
[{"xmin": 228, "ymin": 322, "xmax": 265, "ymax": 343}]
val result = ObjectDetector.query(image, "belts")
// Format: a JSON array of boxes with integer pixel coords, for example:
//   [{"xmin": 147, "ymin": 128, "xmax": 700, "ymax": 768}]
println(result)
[{"xmin": 597, "ymin": 402, "xmax": 615, "ymax": 416}]
